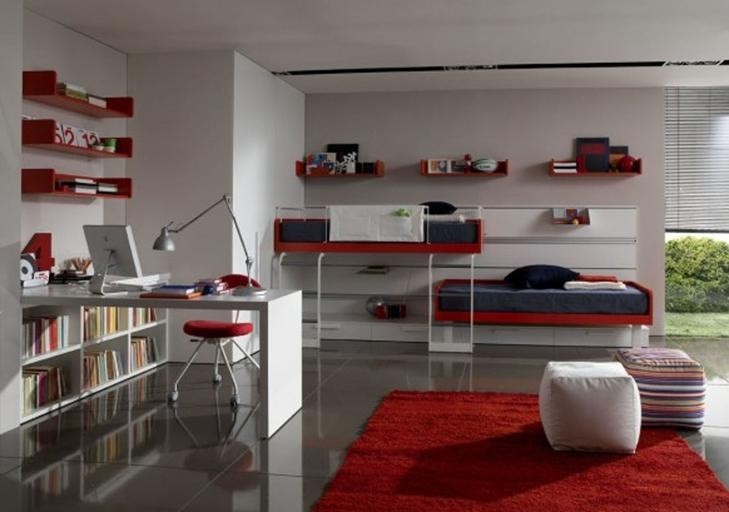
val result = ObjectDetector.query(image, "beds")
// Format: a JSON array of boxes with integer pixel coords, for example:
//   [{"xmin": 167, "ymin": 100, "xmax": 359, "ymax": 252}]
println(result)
[
  {"xmin": 273, "ymin": 204, "xmax": 484, "ymax": 253},
  {"xmin": 433, "ymin": 278, "xmax": 653, "ymax": 325}
]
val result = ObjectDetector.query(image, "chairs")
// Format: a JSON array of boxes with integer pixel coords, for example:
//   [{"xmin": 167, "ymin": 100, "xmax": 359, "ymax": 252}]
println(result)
[{"xmin": 171, "ymin": 272, "xmax": 265, "ymax": 414}]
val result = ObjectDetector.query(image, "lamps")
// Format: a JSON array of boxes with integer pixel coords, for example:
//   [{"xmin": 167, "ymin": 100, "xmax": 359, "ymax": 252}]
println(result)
[{"xmin": 153, "ymin": 193, "xmax": 268, "ymax": 296}]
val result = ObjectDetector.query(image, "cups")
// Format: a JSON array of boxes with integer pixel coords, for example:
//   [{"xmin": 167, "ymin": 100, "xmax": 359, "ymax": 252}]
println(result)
[{"xmin": 91, "ymin": 138, "xmax": 117, "ymax": 153}]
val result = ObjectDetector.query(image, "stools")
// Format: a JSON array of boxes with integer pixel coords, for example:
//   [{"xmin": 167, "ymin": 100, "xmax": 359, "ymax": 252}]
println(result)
[{"xmin": 539, "ymin": 347, "xmax": 707, "ymax": 455}]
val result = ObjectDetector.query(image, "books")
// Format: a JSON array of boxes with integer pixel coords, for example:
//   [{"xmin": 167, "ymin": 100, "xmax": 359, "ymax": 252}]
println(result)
[
  {"xmin": 56, "ymin": 81, "xmax": 107, "ymax": 109},
  {"xmin": 23, "ymin": 305, "xmax": 160, "ymax": 414},
  {"xmin": 55, "ymin": 177, "xmax": 118, "ymax": 195},
  {"xmin": 552, "ymin": 161, "xmax": 579, "ymax": 174},
  {"xmin": 139, "ymin": 278, "xmax": 233, "ymax": 299}
]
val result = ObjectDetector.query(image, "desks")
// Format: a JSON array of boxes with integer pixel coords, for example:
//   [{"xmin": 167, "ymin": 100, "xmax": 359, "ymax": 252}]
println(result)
[{"xmin": 20, "ymin": 279, "xmax": 304, "ymax": 442}]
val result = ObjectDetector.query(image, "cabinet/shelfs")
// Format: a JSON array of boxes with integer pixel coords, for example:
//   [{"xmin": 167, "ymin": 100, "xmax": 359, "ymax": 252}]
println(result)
[
  {"xmin": 22, "ymin": 70, "xmax": 135, "ymax": 198},
  {"xmin": 320, "ymin": 312, "xmax": 650, "ymax": 349},
  {"xmin": 20, "ymin": 304, "xmax": 169, "ymax": 426}
]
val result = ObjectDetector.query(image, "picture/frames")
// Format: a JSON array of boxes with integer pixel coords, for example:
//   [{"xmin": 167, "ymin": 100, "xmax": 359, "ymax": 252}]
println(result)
[{"xmin": 428, "ymin": 158, "xmax": 453, "ymax": 175}]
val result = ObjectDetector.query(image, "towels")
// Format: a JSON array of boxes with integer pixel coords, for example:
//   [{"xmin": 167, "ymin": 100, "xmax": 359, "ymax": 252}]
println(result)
[{"xmin": 563, "ymin": 274, "xmax": 627, "ymax": 291}]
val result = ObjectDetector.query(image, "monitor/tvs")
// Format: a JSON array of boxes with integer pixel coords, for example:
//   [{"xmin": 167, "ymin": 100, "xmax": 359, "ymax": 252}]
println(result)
[{"xmin": 82, "ymin": 224, "xmax": 143, "ymax": 296}]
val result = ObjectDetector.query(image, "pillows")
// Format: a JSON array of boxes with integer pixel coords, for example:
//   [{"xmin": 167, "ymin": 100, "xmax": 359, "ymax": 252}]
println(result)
[
  {"xmin": 503, "ymin": 264, "xmax": 580, "ymax": 290},
  {"xmin": 417, "ymin": 199, "xmax": 457, "ymax": 214}
]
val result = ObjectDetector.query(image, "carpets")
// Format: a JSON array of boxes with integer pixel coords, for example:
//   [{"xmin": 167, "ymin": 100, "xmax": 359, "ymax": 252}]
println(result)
[{"xmin": 311, "ymin": 391, "xmax": 727, "ymax": 512}]
